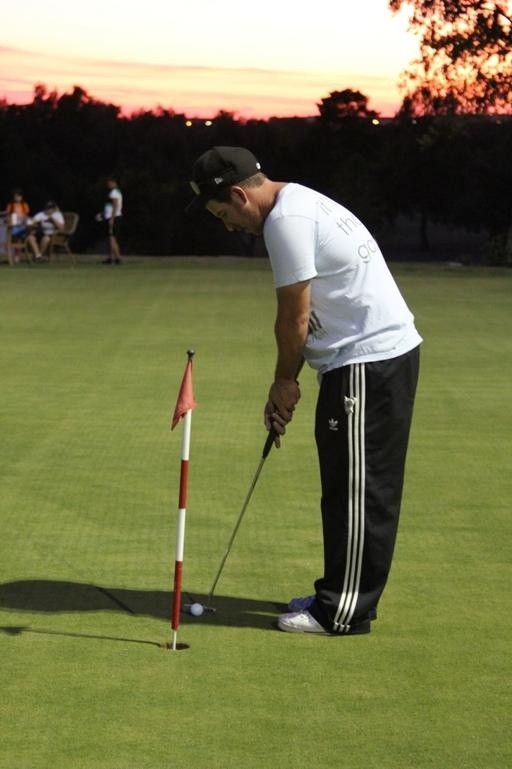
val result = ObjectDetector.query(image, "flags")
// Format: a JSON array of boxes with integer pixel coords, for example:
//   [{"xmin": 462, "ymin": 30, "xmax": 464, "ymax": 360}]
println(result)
[{"xmin": 171, "ymin": 360, "xmax": 198, "ymax": 433}]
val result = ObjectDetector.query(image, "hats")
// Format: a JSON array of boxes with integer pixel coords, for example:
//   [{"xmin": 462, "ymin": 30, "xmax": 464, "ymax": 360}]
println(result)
[{"xmin": 181, "ymin": 145, "xmax": 263, "ymax": 215}]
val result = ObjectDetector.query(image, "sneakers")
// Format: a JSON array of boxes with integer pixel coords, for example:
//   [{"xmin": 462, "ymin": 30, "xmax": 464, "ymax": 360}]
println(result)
[
  {"xmin": 103, "ymin": 258, "xmax": 123, "ymax": 264},
  {"xmin": 35, "ymin": 256, "xmax": 48, "ymax": 263},
  {"xmin": 277, "ymin": 609, "xmax": 332, "ymax": 635},
  {"xmin": 288, "ymin": 595, "xmax": 316, "ymax": 611}
]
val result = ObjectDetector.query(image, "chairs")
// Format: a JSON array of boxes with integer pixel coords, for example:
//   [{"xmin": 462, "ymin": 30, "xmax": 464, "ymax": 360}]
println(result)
[{"xmin": 0, "ymin": 212, "xmax": 80, "ymax": 266}]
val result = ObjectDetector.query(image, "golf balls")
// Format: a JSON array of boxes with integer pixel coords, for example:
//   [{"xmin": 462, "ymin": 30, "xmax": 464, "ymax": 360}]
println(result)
[{"xmin": 191, "ymin": 603, "xmax": 203, "ymax": 616}]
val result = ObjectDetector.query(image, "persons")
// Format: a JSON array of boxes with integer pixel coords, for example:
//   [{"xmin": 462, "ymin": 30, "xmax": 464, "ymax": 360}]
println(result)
[
  {"xmin": 28, "ymin": 199, "xmax": 66, "ymax": 262},
  {"xmin": 0, "ymin": 191, "xmax": 30, "ymax": 264},
  {"xmin": 101, "ymin": 179, "xmax": 123, "ymax": 265},
  {"xmin": 190, "ymin": 145, "xmax": 423, "ymax": 632}
]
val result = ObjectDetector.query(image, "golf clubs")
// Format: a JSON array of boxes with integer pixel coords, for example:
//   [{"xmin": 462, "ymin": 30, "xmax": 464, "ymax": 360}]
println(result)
[{"xmin": 183, "ymin": 416, "xmax": 276, "ymax": 613}]
